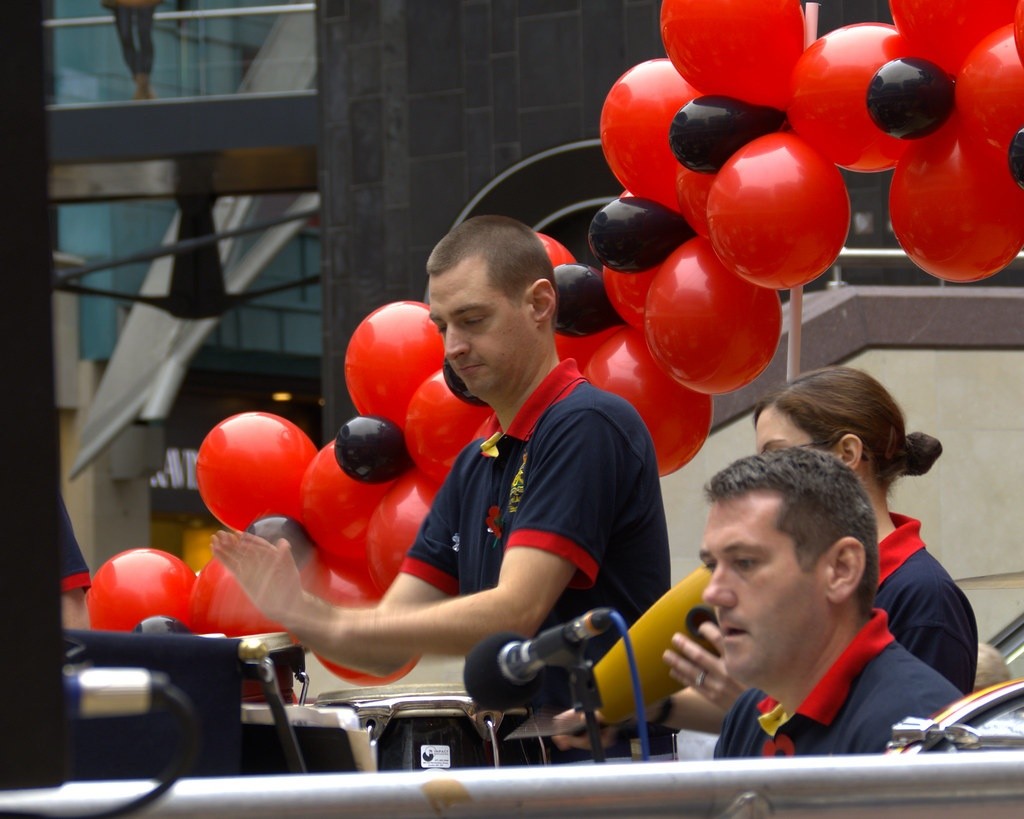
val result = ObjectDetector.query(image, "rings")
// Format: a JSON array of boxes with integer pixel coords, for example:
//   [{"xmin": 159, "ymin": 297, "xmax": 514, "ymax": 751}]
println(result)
[{"xmin": 695, "ymin": 670, "xmax": 707, "ymax": 689}]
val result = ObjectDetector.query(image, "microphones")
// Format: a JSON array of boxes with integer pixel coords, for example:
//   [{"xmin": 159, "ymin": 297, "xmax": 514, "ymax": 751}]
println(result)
[{"xmin": 464, "ymin": 608, "xmax": 614, "ymax": 711}]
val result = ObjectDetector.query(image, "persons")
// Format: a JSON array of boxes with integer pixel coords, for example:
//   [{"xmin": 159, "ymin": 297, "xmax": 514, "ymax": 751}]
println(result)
[
  {"xmin": 701, "ymin": 449, "xmax": 966, "ymax": 758},
  {"xmin": 102, "ymin": 0, "xmax": 163, "ymax": 101},
  {"xmin": 973, "ymin": 642, "xmax": 1011, "ymax": 694},
  {"xmin": 663, "ymin": 367, "xmax": 978, "ymax": 695},
  {"xmin": 60, "ymin": 492, "xmax": 91, "ymax": 631},
  {"xmin": 210, "ymin": 215, "xmax": 679, "ymax": 766}
]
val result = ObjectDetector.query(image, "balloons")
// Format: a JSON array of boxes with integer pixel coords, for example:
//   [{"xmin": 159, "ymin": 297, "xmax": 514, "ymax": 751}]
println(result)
[
  {"xmin": 535, "ymin": 1, "xmax": 1024, "ymax": 478},
  {"xmin": 89, "ymin": 303, "xmax": 496, "ymax": 705}
]
